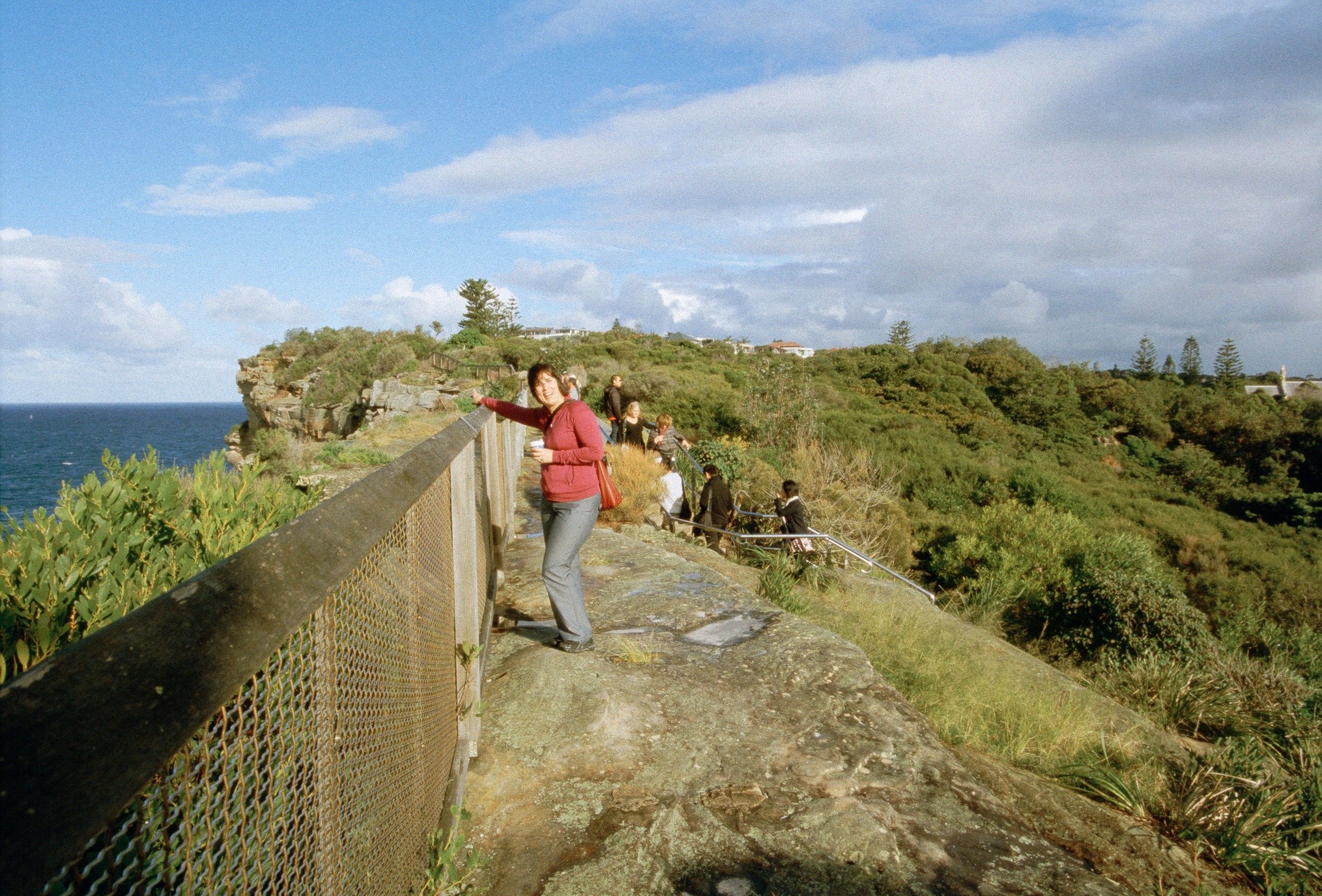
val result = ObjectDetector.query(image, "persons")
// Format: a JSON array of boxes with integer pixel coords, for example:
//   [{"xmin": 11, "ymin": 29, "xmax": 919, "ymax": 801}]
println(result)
[
  {"xmin": 472, "ymin": 364, "xmax": 604, "ymax": 654},
  {"xmin": 561, "ymin": 370, "xmax": 816, "ymax": 556}
]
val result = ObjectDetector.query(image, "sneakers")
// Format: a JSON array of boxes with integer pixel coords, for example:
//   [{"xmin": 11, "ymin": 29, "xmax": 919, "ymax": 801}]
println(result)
[
  {"xmin": 551, "ymin": 636, "xmax": 562, "ymax": 646},
  {"xmin": 559, "ymin": 638, "xmax": 595, "ymax": 653}
]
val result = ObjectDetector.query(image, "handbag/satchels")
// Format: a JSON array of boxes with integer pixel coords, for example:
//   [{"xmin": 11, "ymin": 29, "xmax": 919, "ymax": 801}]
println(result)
[
  {"xmin": 678, "ymin": 498, "xmax": 691, "ymax": 524},
  {"xmin": 596, "ymin": 461, "xmax": 623, "ymax": 512},
  {"xmin": 790, "ymin": 537, "xmax": 815, "ymax": 555},
  {"xmin": 693, "ymin": 512, "xmax": 713, "ymax": 537}
]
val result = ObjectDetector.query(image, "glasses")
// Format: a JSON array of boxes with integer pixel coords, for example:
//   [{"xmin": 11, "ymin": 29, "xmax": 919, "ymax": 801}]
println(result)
[{"xmin": 616, "ymin": 380, "xmax": 621, "ymax": 382}]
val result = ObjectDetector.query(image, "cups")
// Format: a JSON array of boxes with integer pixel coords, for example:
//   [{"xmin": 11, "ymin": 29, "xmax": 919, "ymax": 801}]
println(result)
[{"xmin": 531, "ymin": 439, "xmax": 545, "ymax": 450}]
